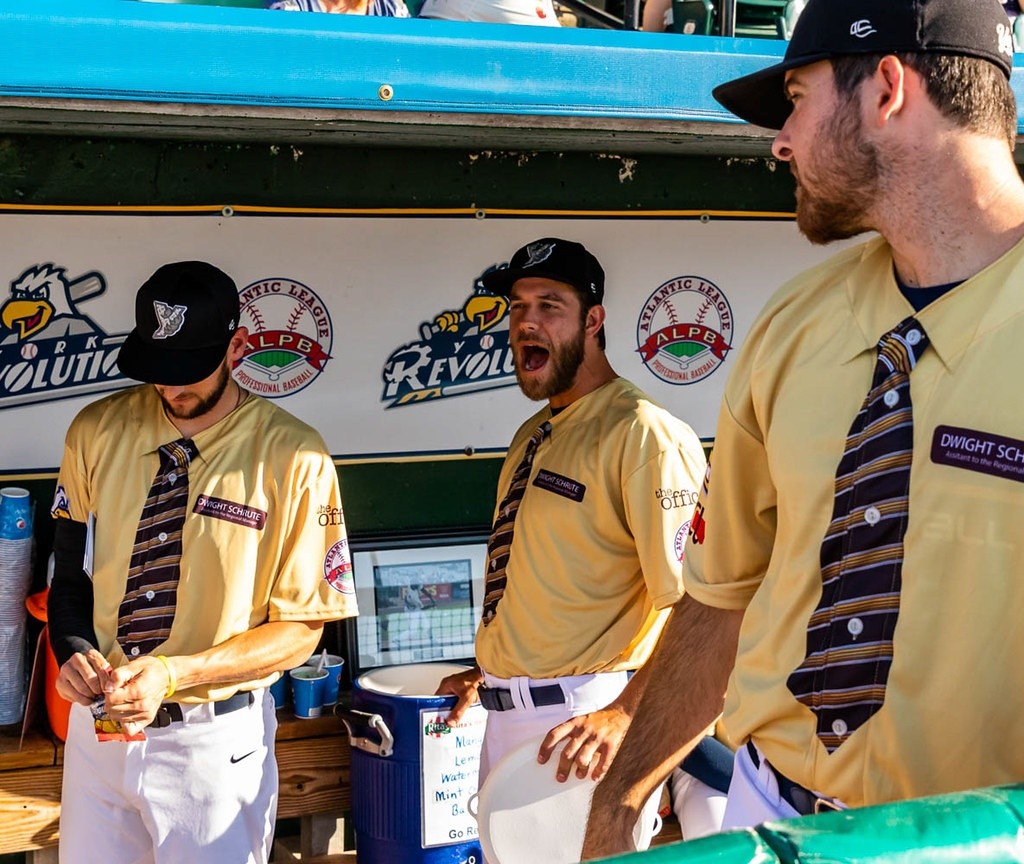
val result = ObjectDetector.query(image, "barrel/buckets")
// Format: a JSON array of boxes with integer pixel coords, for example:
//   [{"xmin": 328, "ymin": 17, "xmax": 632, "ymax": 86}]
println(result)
[{"xmin": 332, "ymin": 662, "xmax": 488, "ymax": 864}]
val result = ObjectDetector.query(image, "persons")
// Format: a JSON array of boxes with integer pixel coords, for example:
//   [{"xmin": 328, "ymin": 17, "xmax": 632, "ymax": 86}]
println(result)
[
  {"xmin": 580, "ymin": 0, "xmax": 1024, "ymax": 860},
  {"xmin": 419, "ymin": 0, "xmax": 562, "ymax": 27},
  {"xmin": 47, "ymin": 260, "xmax": 359, "ymax": 864},
  {"xmin": 436, "ymin": 237, "xmax": 707, "ymax": 792},
  {"xmin": 270, "ymin": 0, "xmax": 411, "ymax": 18},
  {"xmin": 643, "ymin": 0, "xmax": 672, "ymax": 31}
]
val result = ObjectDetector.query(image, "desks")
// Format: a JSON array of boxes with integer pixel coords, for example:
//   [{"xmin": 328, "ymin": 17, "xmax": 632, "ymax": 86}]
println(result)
[{"xmin": 0, "ymin": 707, "xmax": 357, "ymax": 864}]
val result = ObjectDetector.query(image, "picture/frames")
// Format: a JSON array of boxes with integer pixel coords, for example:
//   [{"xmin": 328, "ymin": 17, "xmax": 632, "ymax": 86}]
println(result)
[{"xmin": 340, "ymin": 530, "xmax": 501, "ymax": 676}]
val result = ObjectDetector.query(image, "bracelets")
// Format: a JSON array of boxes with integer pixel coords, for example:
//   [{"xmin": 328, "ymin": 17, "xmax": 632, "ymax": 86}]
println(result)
[{"xmin": 158, "ymin": 655, "xmax": 176, "ymax": 699}]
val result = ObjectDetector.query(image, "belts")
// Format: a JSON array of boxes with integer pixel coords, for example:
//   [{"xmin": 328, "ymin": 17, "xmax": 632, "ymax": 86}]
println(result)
[
  {"xmin": 747, "ymin": 741, "xmax": 839, "ymax": 817},
  {"xmin": 477, "ymin": 670, "xmax": 636, "ymax": 713},
  {"xmin": 91, "ymin": 686, "xmax": 255, "ymax": 728}
]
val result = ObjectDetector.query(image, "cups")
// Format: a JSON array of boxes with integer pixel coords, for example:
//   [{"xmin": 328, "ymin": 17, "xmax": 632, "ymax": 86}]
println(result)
[
  {"xmin": 270, "ymin": 676, "xmax": 288, "ymax": 708},
  {"xmin": 306, "ymin": 654, "xmax": 344, "ymax": 705},
  {"xmin": 0, "ymin": 486, "xmax": 34, "ymax": 725},
  {"xmin": 289, "ymin": 666, "xmax": 329, "ymax": 719}
]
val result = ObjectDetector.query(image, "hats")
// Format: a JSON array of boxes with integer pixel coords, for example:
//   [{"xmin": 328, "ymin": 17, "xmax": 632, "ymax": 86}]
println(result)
[
  {"xmin": 117, "ymin": 260, "xmax": 239, "ymax": 386},
  {"xmin": 719, "ymin": 0, "xmax": 1014, "ymax": 130},
  {"xmin": 481, "ymin": 238, "xmax": 605, "ymax": 302}
]
